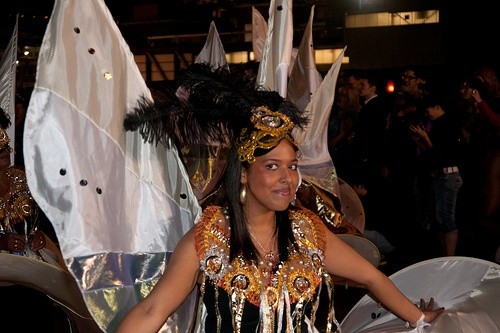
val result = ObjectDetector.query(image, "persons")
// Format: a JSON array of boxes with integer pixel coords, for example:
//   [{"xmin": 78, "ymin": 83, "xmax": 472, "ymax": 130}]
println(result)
[
  {"xmin": 316, "ymin": 49, "xmax": 500, "ymax": 273},
  {"xmin": 111, "ymin": 113, "xmax": 448, "ymax": 333},
  {"xmin": 0, "ymin": 136, "xmax": 58, "ymax": 270}
]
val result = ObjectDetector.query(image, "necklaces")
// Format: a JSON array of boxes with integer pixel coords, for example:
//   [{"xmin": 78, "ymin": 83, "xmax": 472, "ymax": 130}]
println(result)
[{"xmin": 221, "ymin": 202, "xmax": 303, "ymax": 299}]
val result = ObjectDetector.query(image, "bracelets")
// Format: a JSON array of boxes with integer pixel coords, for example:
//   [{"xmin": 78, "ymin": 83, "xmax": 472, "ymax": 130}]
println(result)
[{"xmin": 404, "ymin": 310, "xmax": 426, "ymax": 329}]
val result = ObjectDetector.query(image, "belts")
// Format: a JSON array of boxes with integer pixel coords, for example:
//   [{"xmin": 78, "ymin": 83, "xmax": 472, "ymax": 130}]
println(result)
[{"xmin": 442, "ymin": 166, "xmax": 458, "ymax": 173}]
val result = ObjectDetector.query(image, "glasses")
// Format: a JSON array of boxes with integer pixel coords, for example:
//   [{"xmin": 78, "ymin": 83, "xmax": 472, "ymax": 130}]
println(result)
[{"xmin": 402, "ymin": 75, "xmax": 417, "ymax": 80}]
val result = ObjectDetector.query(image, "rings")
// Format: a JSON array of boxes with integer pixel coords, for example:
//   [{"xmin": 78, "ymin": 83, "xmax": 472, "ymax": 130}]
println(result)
[
  {"xmin": 15, "ymin": 240, "xmax": 18, "ymax": 243},
  {"xmin": 14, "ymin": 245, "xmax": 18, "ymax": 248},
  {"xmin": 40, "ymin": 240, "xmax": 43, "ymax": 244}
]
